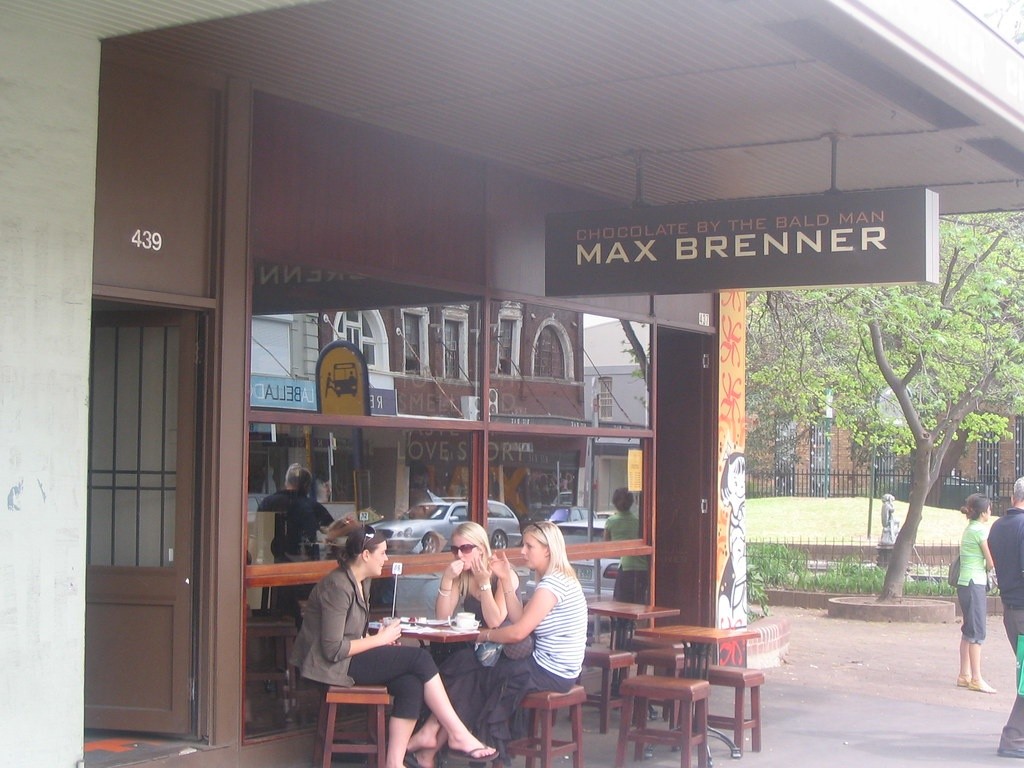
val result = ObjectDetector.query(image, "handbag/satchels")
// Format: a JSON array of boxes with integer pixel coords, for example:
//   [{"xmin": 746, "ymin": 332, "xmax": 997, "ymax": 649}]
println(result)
[
  {"xmin": 502, "ymin": 634, "xmax": 534, "ymax": 660},
  {"xmin": 1015, "ymin": 634, "xmax": 1024, "ymax": 697},
  {"xmin": 476, "ymin": 642, "xmax": 502, "ymax": 667},
  {"xmin": 948, "ymin": 560, "xmax": 996, "ymax": 592}
]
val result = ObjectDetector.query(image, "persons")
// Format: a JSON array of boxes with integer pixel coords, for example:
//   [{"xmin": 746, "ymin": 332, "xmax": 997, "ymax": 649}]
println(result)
[
  {"xmin": 287, "ymin": 524, "xmax": 500, "ymax": 768},
  {"xmin": 987, "ymin": 475, "xmax": 1024, "ymax": 759},
  {"xmin": 956, "ymin": 492, "xmax": 998, "ymax": 694},
  {"xmin": 600, "ymin": 488, "xmax": 650, "ymax": 650},
  {"xmin": 402, "ymin": 521, "xmax": 589, "ymax": 768},
  {"xmin": 255, "ymin": 462, "xmax": 358, "ymax": 702}
]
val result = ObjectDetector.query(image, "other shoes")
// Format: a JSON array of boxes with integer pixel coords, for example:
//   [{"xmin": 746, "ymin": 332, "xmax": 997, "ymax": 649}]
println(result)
[
  {"xmin": 403, "ymin": 750, "xmax": 425, "ymax": 768},
  {"xmin": 998, "ymin": 748, "xmax": 1024, "ymax": 759}
]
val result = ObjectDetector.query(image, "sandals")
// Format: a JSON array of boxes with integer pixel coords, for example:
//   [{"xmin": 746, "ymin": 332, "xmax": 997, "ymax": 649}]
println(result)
[{"xmin": 957, "ymin": 675, "xmax": 996, "ymax": 693}]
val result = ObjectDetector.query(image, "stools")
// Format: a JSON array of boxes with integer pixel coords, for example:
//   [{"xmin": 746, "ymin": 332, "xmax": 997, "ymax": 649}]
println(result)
[
  {"xmin": 316, "ymin": 682, "xmax": 391, "ymax": 768},
  {"xmin": 494, "ymin": 635, "xmax": 765, "ymax": 768},
  {"xmin": 246, "ymin": 606, "xmax": 297, "ymax": 724}
]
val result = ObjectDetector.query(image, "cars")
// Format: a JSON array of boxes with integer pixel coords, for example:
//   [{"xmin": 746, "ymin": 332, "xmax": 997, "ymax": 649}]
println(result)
[
  {"xmin": 526, "ymin": 506, "xmax": 596, "ymax": 526},
  {"xmin": 367, "ymin": 499, "xmax": 523, "ymax": 553},
  {"xmin": 526, "ymin": 523, "xmax": 622, "ymax": 620},
  {"xmin": 246, "ymin": 493, "xmax": 272, "ymax": 524},
  {"xmin": 551, "ymin": 492, "xmax": 574, "ymax": 514},
  {"xmin": 596, "ymin": 510, "xmax": 618, "ymax": 519}
]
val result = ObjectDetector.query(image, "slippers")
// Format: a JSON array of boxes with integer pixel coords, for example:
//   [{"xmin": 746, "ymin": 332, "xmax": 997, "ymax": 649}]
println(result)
[{"xmin": 447, "ymin": 741, "xmax": 500, "ymax": 763}]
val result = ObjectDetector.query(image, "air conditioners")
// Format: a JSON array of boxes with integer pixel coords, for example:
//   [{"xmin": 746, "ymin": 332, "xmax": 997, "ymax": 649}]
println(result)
[
  {"xmin": 489, "ymin": 388, "xmax": 499, "ymax": 413},
  {"xmin": 462, "ymin": 396, "xmax": 480, "ymax": 420}
]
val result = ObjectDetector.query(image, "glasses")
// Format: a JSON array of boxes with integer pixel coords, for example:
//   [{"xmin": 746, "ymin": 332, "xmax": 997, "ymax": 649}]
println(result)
[
  {"xmin": 361, "ymin": 524, "xmax": 375, "ymax": 549},
  {"xmin": 449, "ymin": 544, "xmax": 478, "ymax": 554}
]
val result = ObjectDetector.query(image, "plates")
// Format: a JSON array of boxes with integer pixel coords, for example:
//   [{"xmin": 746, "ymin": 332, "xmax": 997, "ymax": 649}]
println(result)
[{"xmin": 450, "ymin": 626, "xmax": 478, "ymax": 633}]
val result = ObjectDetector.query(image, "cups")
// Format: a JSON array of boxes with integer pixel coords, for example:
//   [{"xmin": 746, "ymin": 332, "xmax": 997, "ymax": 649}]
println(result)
[
  {"xmin": 456, "ymin": 612, "xmax": 479, "ymax": 629},
  {"xmin": 382, "ymin": 616, "xmax": 398, "ymax": 629}
]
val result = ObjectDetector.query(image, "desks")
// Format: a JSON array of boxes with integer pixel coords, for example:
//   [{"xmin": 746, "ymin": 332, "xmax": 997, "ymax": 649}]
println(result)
[
  {"xmin": 587, "ymin": 600, "xmax": 681, "ymax": 719},
  {"xmin": 633, "ymin": 625, "xmax": 761, "ymax": 768},
  {"xmin": 370, "ymin": 617, "xmax": 479, "ymax": 645}
]
